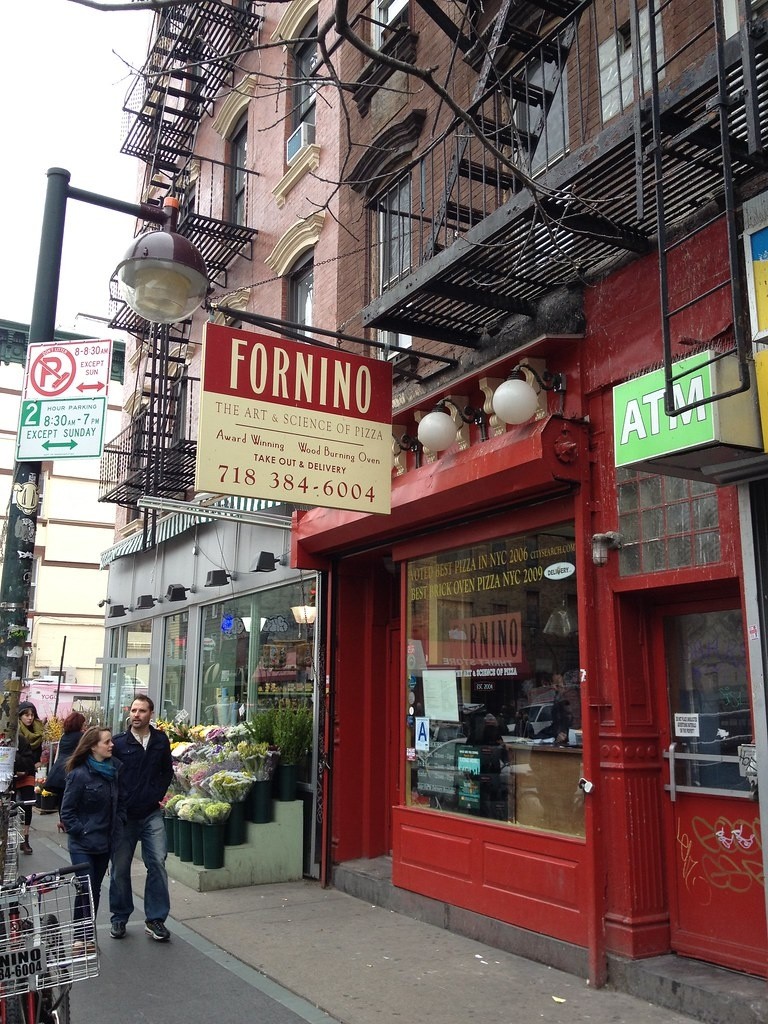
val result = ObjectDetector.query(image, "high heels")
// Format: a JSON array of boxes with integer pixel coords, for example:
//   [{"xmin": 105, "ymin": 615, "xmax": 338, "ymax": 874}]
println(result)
[{"xmin": 57, "ymin": 822, "xmax": 64, "ymax": 833}]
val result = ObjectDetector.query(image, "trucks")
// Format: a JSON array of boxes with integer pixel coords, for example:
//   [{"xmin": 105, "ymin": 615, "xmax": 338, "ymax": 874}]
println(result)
[{"xmin": 19, "ymin": 677, "xmax": 148, "ymax": 722}]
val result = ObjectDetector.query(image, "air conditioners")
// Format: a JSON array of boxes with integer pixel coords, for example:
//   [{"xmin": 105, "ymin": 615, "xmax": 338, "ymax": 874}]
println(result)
[{"xmin": 285, "ymin": 121, "xmax": 314, "ymax": 166}]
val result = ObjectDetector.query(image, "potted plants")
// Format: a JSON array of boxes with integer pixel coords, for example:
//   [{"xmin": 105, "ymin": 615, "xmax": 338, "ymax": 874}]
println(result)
[{"xmin": 270, "ymin": 697, "xmax": 313, "ymax": 803}]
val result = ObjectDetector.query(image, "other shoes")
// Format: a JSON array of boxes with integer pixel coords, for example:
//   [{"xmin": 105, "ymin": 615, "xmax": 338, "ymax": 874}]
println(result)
[
  {"xmin": 84, "ymin": 940, "xmax": 101, "ymax": 956},
  {"xmin": 71, "ymin": 941, "xmax": 85, "ymax": 957},
  {"xmin": 20, "ymin": 841, "xmax": 32, "ymax": 854}
]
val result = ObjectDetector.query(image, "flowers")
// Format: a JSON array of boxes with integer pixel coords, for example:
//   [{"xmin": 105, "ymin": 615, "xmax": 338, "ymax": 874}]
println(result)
[
  {"xmin": 33, "ymin": 778, "xmax": 58, "ymax": 797},
  {"xmin": 143, "ymin": 717, "xmax": 275, "ymax": 822}
]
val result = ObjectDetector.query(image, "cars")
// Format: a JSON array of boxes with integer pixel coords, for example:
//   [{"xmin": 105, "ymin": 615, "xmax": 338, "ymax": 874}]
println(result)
[
  {"xmin": 203, "ymin": 695, "xmax": 248, "ymax": 724},
  {"xmin": 415, "ymin": 721, "xmax": 532, "ymax": 812},
  {"xmin": 507, "ymin": 704, "xmax": 556, "ymax": 735},
  {"xmin": 162, "ymin": 699, "xmax": 176, "ymax": 720}
]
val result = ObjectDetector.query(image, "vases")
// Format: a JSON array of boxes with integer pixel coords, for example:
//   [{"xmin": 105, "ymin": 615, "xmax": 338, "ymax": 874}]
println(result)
[
  {"xmin": 34, "ymin": 793, "xmax": 41, "ymax": 807},
  {"xmin": 157, "ymin": 780, "xmax": 271, "ymax": 869},
  {"xmin": 41, "ymin": 796, "xmax": 55, "ymax": 809}
]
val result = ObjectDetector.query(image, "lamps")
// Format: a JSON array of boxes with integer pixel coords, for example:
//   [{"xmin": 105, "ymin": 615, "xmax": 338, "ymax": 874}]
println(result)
[
  {"xmin": 492, "ymin": 362, "xmax": 565, "ymax": 426},
  {"xmin": 591, "ymin": 530, "xmax": 625, "ymax": 568},
  {"xmin": 238, "ymin": 617, "xmax": 267, "ymax": 632},
  {"xmin": 136, "ymin": 594, "xmax": 164, "ymax": 610},
  {"xmin": 108, "ymin": 604, "xmax": 134, "ymax": 618},
  {"xmin": 290, "ymin": 588, "xmax": 316, "ymax": 624},
  {"xmin": 254, "ymin": 550, "xmax": 288, "ymax": 573},
  {"xmin": 204, "ymin": 570, "xmax": 238, "ymax": 588},
  {"xmin": 416, "ymin": 397, "xmax": 487, "ymax": 452},
  {"xmin": 165, "ymin": 583, "xmax": 196, "ymax": 603}
]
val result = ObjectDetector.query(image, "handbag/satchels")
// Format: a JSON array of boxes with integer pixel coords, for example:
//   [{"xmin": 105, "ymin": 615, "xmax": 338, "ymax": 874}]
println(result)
[{"xmin": 43, "ymin": 755, "xmax": 71, "ymax": 794}]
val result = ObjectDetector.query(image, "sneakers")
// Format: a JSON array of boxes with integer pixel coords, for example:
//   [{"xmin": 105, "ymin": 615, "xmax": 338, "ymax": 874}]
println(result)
[
  {"xmin": 144, "ymin": 920, "xmax": 170, "ymax": 940},
  {"xmin": 109, "ymin": 922, "xmax": 127, "ymax": 939}
]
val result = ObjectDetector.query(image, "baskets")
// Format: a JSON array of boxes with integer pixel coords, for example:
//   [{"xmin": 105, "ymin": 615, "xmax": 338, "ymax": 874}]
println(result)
[
  {"xmin": 2, "ymin": 828, "xmax": 19, "ymax": 885},
  {"xmin": 6, "ymin": 800, "xmax": 25, "ymax": 844},
  {"xmin": 0, "ymin": 874, "xmax": 101, "ymax": 999}
]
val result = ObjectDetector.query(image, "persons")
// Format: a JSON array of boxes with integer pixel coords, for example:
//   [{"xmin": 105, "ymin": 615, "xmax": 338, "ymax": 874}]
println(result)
[
  {"xmin": 11, "ymin": 701, "xmax": 44, "ymax": 855},
  {"xmin": 57, "ymin": 713, "xmax": 85, "ymax": 833},
  {"xmin": 61, "ymin": 694, "xmax": 187, "ymax": 958}
]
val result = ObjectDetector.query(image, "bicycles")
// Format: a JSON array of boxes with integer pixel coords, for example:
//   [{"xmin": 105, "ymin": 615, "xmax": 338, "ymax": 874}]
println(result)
[{"xmin": 0, "ymin": 799, "xmax": 102, "ymax": 1024}]
